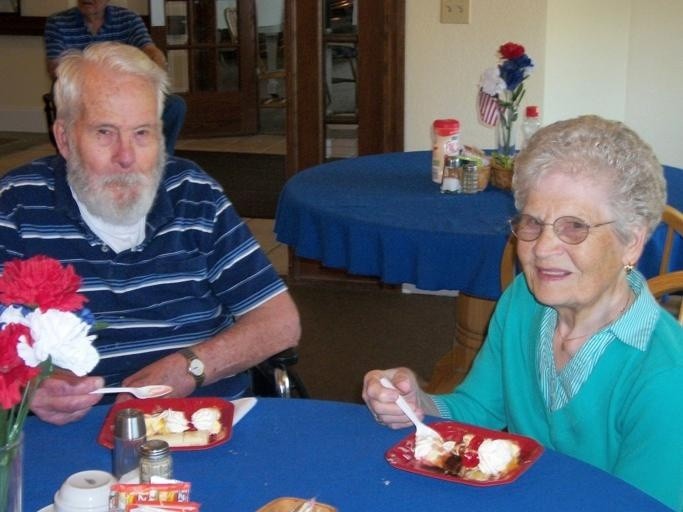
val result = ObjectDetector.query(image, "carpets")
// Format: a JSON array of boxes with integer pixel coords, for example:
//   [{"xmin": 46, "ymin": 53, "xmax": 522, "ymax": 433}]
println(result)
[{"xmin": 169, "ymin": 147, "xmax": 349, "ymax": 221}]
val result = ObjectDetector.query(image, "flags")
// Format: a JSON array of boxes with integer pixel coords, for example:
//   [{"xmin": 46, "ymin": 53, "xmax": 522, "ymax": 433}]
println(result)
[{"xmin": 479, "ymin": 88, "xmax": 506, "ymax": 126}]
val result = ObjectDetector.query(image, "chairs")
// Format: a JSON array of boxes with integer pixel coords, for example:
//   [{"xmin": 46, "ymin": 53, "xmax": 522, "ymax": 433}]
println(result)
[{"xmin": 495, "ymin": 203, "xmax": 683, "ymax": 322}]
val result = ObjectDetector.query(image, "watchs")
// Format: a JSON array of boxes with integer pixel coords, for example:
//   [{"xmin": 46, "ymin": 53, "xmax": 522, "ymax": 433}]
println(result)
[{"xmin": 178, "ymin": 348, "xmax": 205, "ymax": 392}]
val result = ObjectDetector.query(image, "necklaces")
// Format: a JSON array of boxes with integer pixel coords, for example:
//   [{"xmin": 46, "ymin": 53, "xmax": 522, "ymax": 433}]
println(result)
[{"xmin": 557, "ymin": 288, "xmax": 631, "ymax": 350}]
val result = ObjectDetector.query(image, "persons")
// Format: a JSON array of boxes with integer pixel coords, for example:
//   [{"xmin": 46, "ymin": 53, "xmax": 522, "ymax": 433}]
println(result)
[
  {"xmin": 362, "ymin": 116, "xmax": 683, "ymax": 512},
  {"xmin": 0, "ymin": 40, "xmax": 301, "ymax": 426},
  {"xmin": 44, "ymin": 0, "xmax": 187, "ymax": 154}
]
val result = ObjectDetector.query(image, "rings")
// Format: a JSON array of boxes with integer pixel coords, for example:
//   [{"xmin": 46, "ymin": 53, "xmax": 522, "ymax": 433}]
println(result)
[{"xmin": 373, "ymin": 413, "xmax": 382, "ymax": 423}]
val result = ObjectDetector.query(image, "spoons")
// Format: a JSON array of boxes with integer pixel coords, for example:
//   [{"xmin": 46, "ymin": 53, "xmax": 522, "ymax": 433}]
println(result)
[
  {"xmin": 379, "ymin": 378, "xmax": 442, "ymax": 442},
  {"xmin": 87, "ymin": 385, "xmax": 173, "ymax": 400}
]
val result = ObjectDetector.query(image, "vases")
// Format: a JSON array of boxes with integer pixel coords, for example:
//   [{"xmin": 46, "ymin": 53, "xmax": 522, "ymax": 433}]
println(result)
[
  {"xmin": 0, "ymin": 428, "xmax": 26, "ymax": 511},
  {"xmin": 486, "ymin": 154, "xmax": 513, "ymax": 192}
]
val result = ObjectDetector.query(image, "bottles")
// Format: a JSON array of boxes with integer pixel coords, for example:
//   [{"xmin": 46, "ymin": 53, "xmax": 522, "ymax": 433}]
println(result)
[
  {"xmin": 431, "ymin": 119, "xmax": 491, "ymax": 193},
  {"xmin": 53, "ymin": 471, "xmax": 118, "ymax": 512},
  {"xmin": 113, "ymin": 408, "xmax": 146, "ymax": 478},
  {"xmin": 139, "ymin": 439, "xmax": 173, "ymax": 483},
  {"xmin": 519, "ymin": 106, "xmax": 541, "ymax": 149}
]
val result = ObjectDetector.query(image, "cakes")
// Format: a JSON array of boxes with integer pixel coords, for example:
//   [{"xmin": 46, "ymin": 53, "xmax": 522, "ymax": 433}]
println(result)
[
  {"xmin": 144, "ymin": 408, "xmax": 222, "ymax": 447},
  {"xmin": 416, "ymin": 429, "xmax": 520, "ymax": 482}
]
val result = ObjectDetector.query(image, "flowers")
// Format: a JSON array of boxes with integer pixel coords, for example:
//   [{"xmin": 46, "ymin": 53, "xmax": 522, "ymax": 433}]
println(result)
[
  {"xmin": 0, "ymin": 253, "xmax": 113, "ymax": 512},
  {"xmin": 474, "ymin": 40, "xmax": 536, "ymax": 167}
]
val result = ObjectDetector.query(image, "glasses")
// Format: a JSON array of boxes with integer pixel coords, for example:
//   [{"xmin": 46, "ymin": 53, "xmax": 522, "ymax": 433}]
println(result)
[{"xmin": 506, "ymin": 214, "xmax": 616, "ymax": 244}]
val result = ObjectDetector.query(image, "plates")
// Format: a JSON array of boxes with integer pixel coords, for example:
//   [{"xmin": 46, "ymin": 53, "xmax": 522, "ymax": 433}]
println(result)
[
  {"xmin": 97, "ymin": 398, "xmax": 233, "ymax": 451},
  {"xmin": 385, "ymin": 421, "xmax": 543, "ymax": 487}
]
